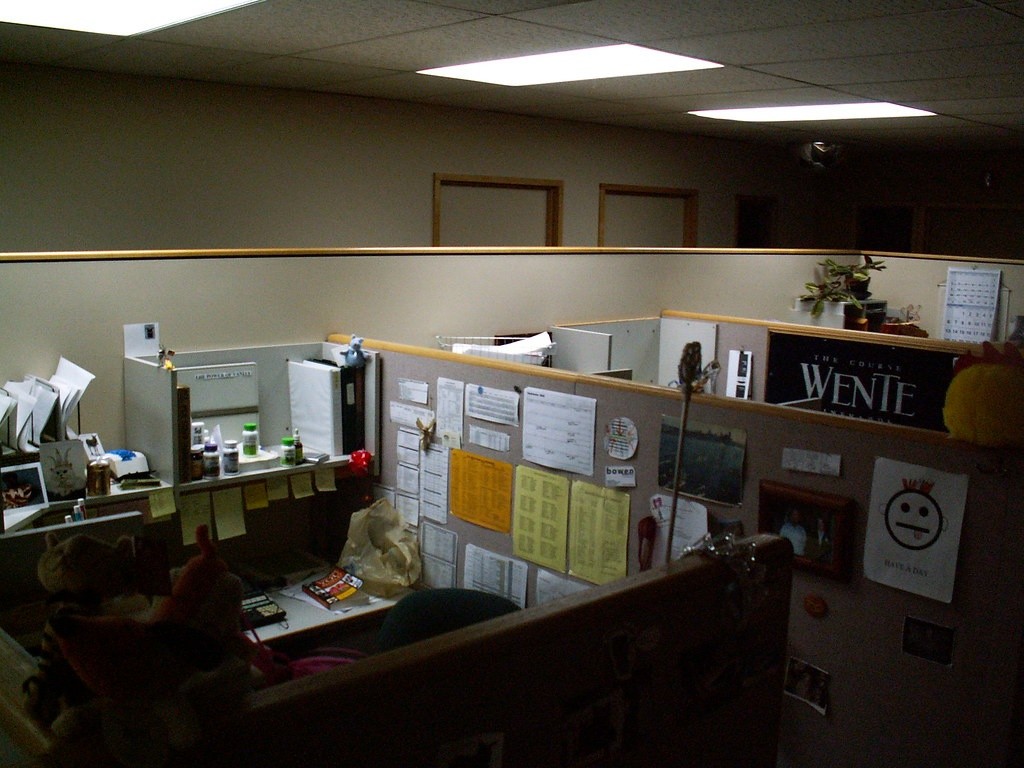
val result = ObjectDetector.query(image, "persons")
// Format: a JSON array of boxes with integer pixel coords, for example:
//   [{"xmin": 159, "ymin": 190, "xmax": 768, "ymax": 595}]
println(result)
[{"xmin": 779, "ymin": 507, "xmax": 831, "ymax": 562}]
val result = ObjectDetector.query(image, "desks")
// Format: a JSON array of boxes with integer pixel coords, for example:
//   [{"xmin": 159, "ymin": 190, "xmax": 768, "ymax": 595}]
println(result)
[{"xmin": 237, "ymin": 566, "xmax": 399, "ymax": 640}]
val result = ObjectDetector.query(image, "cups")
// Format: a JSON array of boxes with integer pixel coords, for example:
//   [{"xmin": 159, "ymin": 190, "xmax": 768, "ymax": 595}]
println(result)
[{"xmin": 86, "ymin": 463, "xmax": 110, "ymax": 496}]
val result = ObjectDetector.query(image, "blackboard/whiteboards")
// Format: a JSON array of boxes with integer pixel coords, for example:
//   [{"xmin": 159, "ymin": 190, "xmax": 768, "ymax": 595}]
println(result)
[{"xmin": 764, "ymin": 327, "xmax": 970, "ymax": 434}]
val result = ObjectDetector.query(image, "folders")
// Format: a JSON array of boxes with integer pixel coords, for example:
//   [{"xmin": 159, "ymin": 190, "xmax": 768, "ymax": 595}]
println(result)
[{"xmin": 289, "ymin": 360, "xmax": 365, "ymax": 456}]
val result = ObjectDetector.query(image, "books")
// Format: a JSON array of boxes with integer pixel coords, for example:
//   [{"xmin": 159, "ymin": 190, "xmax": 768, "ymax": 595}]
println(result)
[{"xmin": 302, "ymin": 566, "xmax": 370, "ymax": 612}]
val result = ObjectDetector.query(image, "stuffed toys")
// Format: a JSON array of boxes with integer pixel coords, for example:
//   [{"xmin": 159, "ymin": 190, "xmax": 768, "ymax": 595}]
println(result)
[
  {"xmin": 24, "ymin": 525, "xmax": 238, "ymax": 714},
  {"xmin": 340, "ymin": 334, "xmax": 371, "ymax": 368}
]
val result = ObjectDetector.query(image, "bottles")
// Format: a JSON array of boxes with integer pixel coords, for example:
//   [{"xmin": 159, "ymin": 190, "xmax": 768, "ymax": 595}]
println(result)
[{"xmin": 191, "ymin": 423, "xmax": 303, "ymax": 480}]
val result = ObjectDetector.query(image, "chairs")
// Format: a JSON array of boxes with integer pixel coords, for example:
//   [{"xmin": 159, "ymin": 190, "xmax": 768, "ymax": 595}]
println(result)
[{"xmin": 369, "ymin": 584, "xmax": 520, "ymax": 656}]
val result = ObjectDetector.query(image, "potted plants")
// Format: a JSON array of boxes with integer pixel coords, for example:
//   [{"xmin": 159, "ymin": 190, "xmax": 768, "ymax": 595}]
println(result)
[{"xmin": 800, "ymin": 254, "xmax": 887, "ymax": 319}]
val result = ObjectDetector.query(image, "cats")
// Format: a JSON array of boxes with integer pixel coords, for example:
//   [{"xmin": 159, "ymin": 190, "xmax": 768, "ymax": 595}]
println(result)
[{"xmin": 49, "ymin": 448, "xmax": 82, "ymax": 494}]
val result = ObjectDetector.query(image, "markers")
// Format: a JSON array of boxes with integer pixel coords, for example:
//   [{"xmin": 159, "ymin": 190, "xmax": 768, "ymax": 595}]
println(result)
[{"xmin": 65, "ymin": 499, "xmax": 86, "ymax": 523}]
